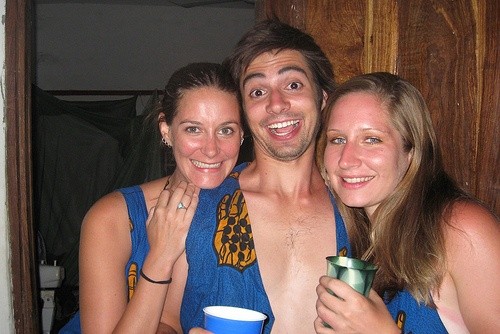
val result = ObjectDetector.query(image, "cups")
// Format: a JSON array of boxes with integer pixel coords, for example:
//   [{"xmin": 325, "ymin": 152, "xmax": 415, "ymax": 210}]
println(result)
[
  {"xmin": 321, "ymin": 256, "xmax": 378, "ymax": 329},
  {"xmin": 200, "ymin": 305, "xmax": 268, "ymax": 334}
]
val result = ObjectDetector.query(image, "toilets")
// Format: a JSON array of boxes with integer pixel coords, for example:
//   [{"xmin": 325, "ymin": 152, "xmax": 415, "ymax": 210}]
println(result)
[{"xmin": 38, "ymin": 266, "xmax": 65, "ymax": 334}]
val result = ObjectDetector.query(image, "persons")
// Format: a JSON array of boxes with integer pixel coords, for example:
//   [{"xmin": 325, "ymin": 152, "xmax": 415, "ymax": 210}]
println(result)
[
  {"xmin": 53, "ymin": 60, "xmax": 246, "ymax": 334},
  {"xmin": 154, "ymin": 16, "xmax": 354, "ymax": 334},
  {"xmin": 313, "ymin": 71, "xmax": 500, "ymax": 334}
]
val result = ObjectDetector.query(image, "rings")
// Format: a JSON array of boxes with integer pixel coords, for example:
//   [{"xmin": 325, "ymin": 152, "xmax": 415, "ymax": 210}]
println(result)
[{"xmin": 176, "ymin": 201, "xmax": 188, "ymax": 210}]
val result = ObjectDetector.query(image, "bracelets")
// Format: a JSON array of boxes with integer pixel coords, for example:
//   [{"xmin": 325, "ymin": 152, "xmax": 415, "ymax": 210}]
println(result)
[{"xmin": 138, "ymin": 270, "xmax": 173, "ymax": 285}]
